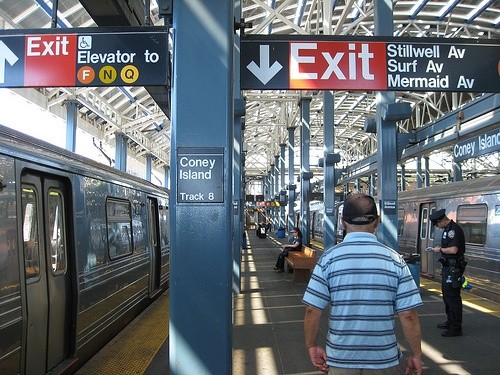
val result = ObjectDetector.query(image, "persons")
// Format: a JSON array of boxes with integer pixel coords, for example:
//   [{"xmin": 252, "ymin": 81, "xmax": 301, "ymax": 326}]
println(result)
[
  {"xmin": 429, "ymin": 209, "xmax": 468, "ymax": 337},
  {"xmin": 273, "ymin": 227, "xmax": 303, "ymax": 273},
  {"xmin": 302, "ymin": 193, "xmax": 424, "ymax": 375}
]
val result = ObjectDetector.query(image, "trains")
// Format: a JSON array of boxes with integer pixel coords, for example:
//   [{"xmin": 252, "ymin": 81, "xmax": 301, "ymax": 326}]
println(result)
[
  {"xmin": 278, "ymin": 92, "xmax": 500, "ymax": 285},
  {"xmin": 0, "ymin": 123, "xmax": 170, "ymax": 375}
]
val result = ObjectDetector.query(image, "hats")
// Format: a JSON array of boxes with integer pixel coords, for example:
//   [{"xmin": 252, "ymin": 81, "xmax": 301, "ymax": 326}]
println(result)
[
  {"xmin": 429, "ymin": 209, "xmax": 446, "ymax": 227},
  {"xmin": 342, "ymin": 194, "xmax": 378, "ymax": 225},
  {"xmin": 289, "ymin": 227, "xmax": 298, "ymax": 232}
]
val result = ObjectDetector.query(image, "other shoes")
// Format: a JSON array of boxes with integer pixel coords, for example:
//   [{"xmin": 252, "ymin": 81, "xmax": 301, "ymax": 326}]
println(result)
[
  {"xmin": 441, "ymin": 327, "xmax": 462, "ymax": 337},
  {"xmin": 275, "ymin": 269, "xmax": 285, "ymax": 273},
  {"xmin": 438, "ymin": 321, "xmax": 449, "ymax": 329},
  {"xmin": 274, "ymin": 267, "xmax": 279, "ymax": 270}
]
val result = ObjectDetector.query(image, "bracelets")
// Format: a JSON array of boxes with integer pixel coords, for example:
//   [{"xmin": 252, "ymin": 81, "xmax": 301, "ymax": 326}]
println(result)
[{"xmin": 440, "ymin": 248, "xmax": 443, "ymax": 253}]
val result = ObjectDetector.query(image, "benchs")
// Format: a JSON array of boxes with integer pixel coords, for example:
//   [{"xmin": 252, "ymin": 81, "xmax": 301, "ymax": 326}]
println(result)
[{"xmin": 284, "ymin": 245, "xmax": 317, "ymax": 283}]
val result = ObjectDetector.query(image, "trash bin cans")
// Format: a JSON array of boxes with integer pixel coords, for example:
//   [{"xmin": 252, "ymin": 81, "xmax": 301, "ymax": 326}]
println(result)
[
  {"xmin": 277, "ymin": 228, "xmax": 285, "ymax": 238},
  {"xmin": 399, "ymin": 251, "xmax": 420, "ymax": 289}
]
